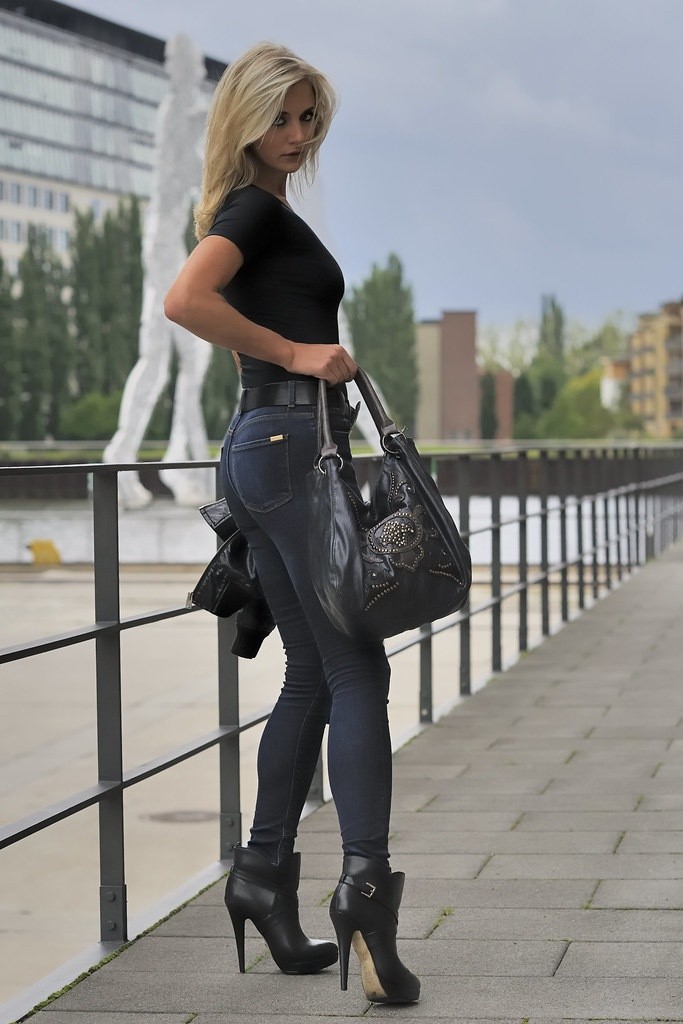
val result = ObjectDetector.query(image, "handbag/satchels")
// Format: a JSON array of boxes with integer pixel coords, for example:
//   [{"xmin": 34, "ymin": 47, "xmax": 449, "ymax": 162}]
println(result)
[{"xmin": 304, "ymin": 365, "xmax": 470, "ymax": 641}]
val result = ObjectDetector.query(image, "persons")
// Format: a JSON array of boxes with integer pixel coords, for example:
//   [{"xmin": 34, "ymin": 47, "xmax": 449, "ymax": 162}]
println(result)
[
  {"xmin": 107, "ymin": 36, "xmax": 212, "ymax": 508},
  {"xmin": 164, "ymin": 45, "xmax": 422, "ymax": 1003}
]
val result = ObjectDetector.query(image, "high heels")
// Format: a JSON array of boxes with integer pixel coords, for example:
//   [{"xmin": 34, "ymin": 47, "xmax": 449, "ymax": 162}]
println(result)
[
  {"xmin": 223, "ymin": 843, "xmax": 337, "ymax": 975},
  {"xmin": 329, "ymin": 851, "xmax": 420, "ymax": 1005}
]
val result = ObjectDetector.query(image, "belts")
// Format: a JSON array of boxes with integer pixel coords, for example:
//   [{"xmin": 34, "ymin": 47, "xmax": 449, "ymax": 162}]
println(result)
[{"xmin": 237, "ymin": 375, "xmax": 344, "ymax": 414}]
